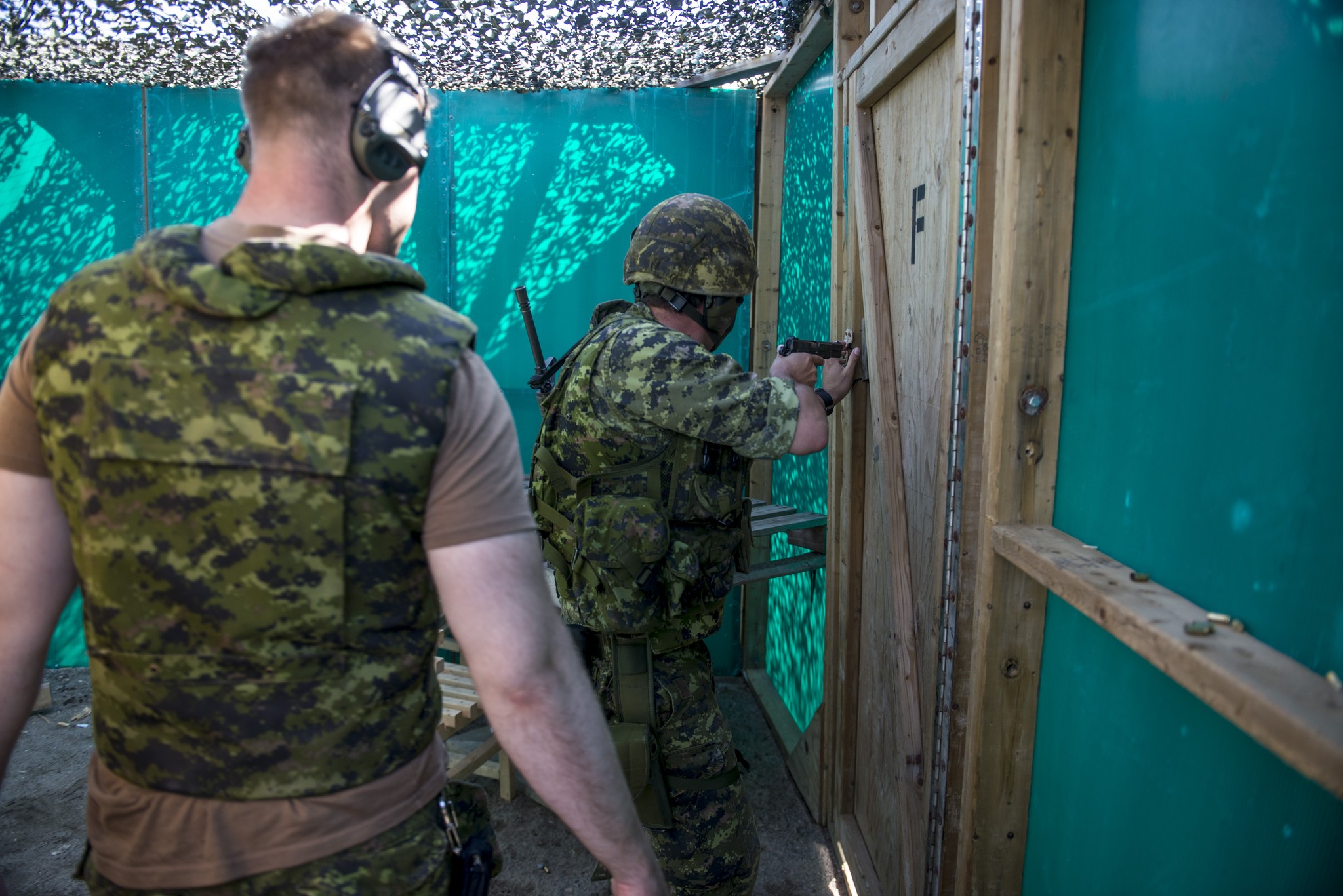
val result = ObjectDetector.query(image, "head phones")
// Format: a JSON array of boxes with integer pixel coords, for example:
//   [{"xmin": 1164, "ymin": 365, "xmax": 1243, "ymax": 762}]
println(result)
[{"xmin": 233, "ymin": 29, "xmax": 429, "ymax": 182}]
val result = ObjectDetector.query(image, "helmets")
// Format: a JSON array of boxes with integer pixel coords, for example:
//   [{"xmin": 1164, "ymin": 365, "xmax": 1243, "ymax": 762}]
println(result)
[{"xmin": 622, "ymin": 192, "xmax": 756, "ymax": 298}]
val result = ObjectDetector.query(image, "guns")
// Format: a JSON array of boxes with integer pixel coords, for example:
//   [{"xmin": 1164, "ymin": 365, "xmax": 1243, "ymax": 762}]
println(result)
[
  {"xmin": 777, "ymin": 335, "xmax": 844, "ymax": 361},
  {"xmin": 512, "ymin": 285, "xmax": 557, "ymax": 416}
]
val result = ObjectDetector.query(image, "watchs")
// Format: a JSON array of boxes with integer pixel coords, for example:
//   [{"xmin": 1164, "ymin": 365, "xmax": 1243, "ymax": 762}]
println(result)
[{"xmin": 813, "ymin": 385, "xmax": 835, "ymax": 418}]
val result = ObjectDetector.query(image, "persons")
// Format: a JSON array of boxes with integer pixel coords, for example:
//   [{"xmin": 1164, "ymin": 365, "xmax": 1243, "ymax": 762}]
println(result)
[
  {"xmin": 2, "ymin": 13, "xmax": 670, "ymax": 896},
  {"xmin": 518, "ymin": 191, "xmax": 861, "ymax": 894}
]
